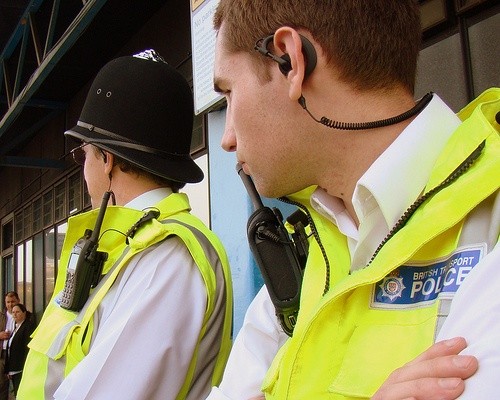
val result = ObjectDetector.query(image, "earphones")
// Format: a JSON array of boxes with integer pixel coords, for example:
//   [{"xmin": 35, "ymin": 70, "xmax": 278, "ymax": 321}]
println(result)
[
  {"xmin": 253, "ymin": 31, "xmax": 319, "ymax": 80},
  {"xmin": 102, "ymin": 151, "xmax": 108, "ymax": 163}
]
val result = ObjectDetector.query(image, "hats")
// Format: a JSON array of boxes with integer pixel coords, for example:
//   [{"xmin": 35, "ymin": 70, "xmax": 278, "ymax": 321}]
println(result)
[{"xmin": 64, "ymin": 49, "xmax": 205, "ymax": 183}]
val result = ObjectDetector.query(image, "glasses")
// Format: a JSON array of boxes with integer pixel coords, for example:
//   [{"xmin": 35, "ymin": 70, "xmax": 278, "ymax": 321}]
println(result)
[{"xmin": 69, "ymin": 142, "xmax": 94, "ymax": 166}]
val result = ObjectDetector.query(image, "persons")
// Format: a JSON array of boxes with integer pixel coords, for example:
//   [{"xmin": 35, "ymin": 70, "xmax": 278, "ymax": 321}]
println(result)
[
  {"xmin": 204, "ymin": 0, "xmax": 500, "ymax": 400},
  {"xmin": 15, "ymin": 49, "xmax": 235, "ymax": 400},
  {"xmin": 5, "ymin": 303, "xmax": 33, "ymax": 397},
  {"xmin": 0, "ymin": 291, "xmax": 20, "ymax": 400}
]
